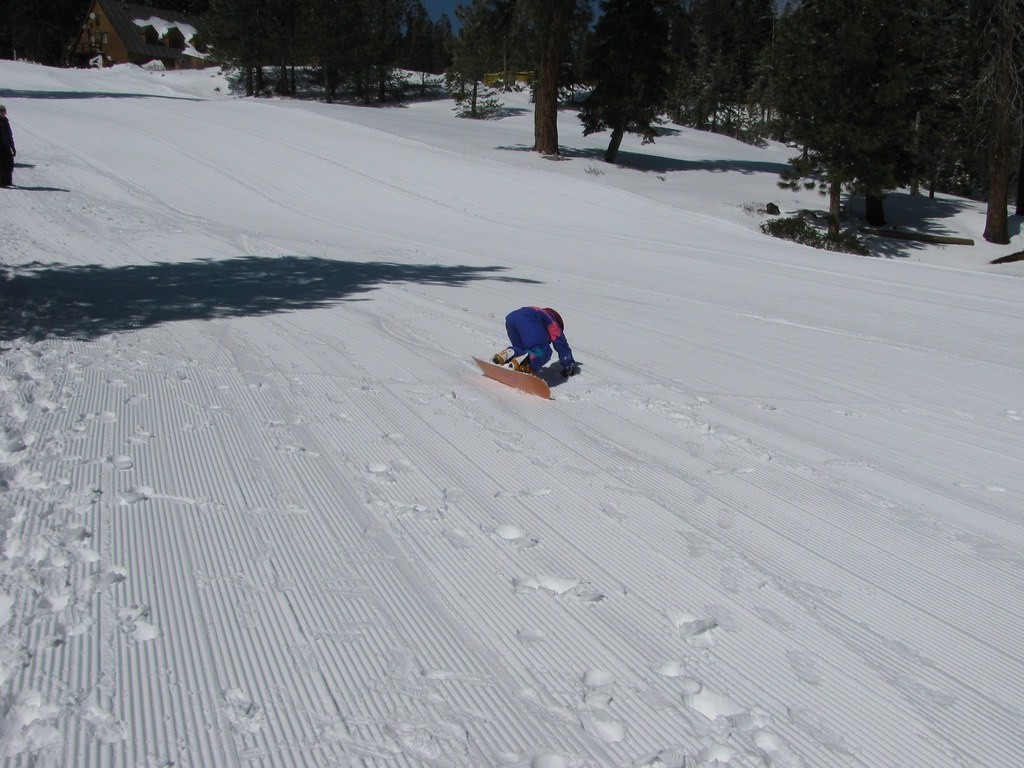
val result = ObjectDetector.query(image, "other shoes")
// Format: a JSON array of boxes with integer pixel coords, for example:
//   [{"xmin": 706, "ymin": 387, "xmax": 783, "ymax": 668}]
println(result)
[
  {"xmin": 508, "ymin": 359, "xmax": 526, "ymax": 371},
  {"xmin": 492, "ymin": 353, "xmax": 507, "ymax": 366}
]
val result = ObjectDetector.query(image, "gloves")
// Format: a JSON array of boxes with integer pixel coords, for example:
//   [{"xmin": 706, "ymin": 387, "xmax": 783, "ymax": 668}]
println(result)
[{"xmin": 561, "ymin": 368, "xmax": 575, "ymax": 376}]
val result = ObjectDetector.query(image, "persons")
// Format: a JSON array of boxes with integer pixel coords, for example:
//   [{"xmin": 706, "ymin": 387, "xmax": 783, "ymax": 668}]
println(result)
[
  {"xmin": 0, "ymin": 104, "xmax": 16, "ymax": 188},
  {"xmin": 492, "ymin": 305, "xmax": 575, "ymax": 377}
]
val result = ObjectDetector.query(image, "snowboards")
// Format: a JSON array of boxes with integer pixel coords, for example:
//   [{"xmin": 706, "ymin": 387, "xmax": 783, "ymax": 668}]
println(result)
[{"xmin": 471, "ymin": 355, "xmax": 550, "ymax": 399}]
